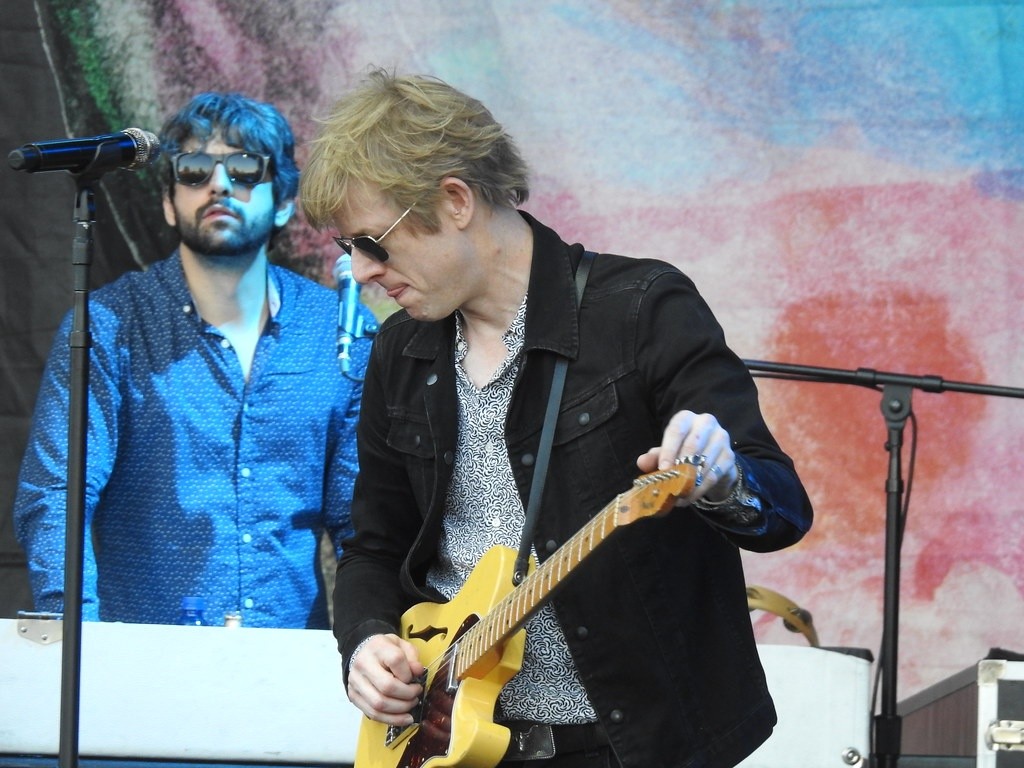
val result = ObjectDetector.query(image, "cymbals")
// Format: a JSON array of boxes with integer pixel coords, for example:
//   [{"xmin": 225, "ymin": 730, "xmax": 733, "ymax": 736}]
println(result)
[{"xmin": 745, "ymin": 582, "xmax": 820, "ymax": 648}]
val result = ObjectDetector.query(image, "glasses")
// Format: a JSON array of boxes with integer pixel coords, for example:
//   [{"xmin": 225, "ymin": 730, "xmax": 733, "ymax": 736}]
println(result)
[
  {"xmin": 169, "ymin": 151, "xmax": 272, "ymax": 185},
  {"xmin": 332, "ymin": 188, "xmax": 431, "ymax": 263}
]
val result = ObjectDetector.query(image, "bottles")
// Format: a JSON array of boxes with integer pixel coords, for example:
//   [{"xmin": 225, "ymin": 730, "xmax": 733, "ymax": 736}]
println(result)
[{"xmin": 177, "ymin": 597, "xmax": 210, "ymax": 626}]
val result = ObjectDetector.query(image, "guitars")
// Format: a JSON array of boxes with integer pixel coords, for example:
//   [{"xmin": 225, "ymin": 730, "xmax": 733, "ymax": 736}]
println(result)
[{"xmin": 350, "ymin": 462, "xmax": 698, "ymax": 768}]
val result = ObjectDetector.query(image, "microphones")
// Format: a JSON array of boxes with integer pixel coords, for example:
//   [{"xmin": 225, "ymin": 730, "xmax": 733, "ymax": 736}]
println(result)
[
  {"xmin": 332, "ymin": 253, "xmax": 365, "ymax": 375},
  {"xmin": 5, "ymin": 127, "xmax": 161, "ymax": 175}
]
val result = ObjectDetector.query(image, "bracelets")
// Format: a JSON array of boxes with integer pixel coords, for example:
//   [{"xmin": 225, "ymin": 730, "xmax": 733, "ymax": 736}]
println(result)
[{"xmin": 698, "ymin": 461, "xmax": 743, "ymax": 506}]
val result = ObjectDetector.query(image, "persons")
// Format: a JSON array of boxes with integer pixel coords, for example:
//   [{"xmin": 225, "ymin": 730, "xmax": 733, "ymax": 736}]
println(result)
[
  {"xmin": 296, "ymin": 64, "xmax": 814, "ymax": 768},
  {"xmin": 14, "ymin": 91, "xmax": 380, "ymax": 631}
]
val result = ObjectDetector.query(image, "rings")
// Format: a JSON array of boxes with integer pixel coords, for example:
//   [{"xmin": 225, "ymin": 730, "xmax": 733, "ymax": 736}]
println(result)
[{"xmin": 675, "ymin": 454, "xmax": 722, "ymax": 486}]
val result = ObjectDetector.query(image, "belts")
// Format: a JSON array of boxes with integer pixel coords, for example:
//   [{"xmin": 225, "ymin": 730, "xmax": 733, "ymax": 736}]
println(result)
[{"xmin": 499, "ymin": 720, "xmax": 612, "ymax": 761}]
